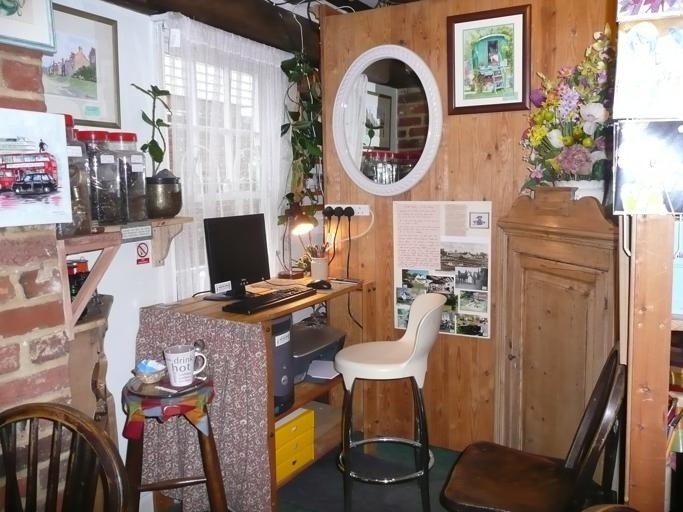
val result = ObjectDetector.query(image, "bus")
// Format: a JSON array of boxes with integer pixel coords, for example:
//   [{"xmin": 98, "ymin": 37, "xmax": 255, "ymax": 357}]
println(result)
[{"xmin": 0, "ymin": 153, "xmax": 58, "ymax": 194}]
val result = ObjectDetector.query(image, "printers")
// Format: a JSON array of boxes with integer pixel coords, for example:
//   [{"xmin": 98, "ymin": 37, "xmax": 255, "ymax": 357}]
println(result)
[{"xmin": 293, "ymin": 318, "xmax": 346, "ymax": 384}]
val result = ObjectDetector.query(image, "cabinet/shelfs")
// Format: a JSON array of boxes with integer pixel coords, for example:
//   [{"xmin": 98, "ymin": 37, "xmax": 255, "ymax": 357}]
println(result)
[{"xmin": 68, "ymin": 290, "xmax": 121, "ymax": 512}]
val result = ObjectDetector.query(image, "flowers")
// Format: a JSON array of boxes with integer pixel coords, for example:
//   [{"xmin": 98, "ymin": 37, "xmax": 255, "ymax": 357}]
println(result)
[{"xmin": 519, "ymin": 20, "xmax": 614, "ymax": 204}]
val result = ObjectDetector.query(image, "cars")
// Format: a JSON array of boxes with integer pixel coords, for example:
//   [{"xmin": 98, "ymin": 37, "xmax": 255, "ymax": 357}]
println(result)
[{"xmin": 14, "ymin": 173, "xmax": 58, "ymax": 196}]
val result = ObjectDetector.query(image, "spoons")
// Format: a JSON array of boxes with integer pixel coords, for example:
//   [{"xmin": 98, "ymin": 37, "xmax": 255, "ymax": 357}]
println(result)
[{"xmin": 192, "ymin": 339, "xmax": 205, "ymax": 364}]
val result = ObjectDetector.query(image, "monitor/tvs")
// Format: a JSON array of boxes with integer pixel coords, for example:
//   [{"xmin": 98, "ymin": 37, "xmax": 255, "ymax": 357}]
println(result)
[{"xmin": 198, "ymin": 212, "xmax": 271, "ymax": 300}]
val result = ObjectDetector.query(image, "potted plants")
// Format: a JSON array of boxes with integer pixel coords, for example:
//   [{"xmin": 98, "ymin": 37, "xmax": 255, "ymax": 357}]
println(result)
[{"xmin": 128, "ymin": 80, "xmax": 182, "ymax": 217}]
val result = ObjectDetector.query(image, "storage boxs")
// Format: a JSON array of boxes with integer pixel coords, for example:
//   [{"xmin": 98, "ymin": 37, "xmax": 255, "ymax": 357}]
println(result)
[{"xmin": 274, "ymin": 408, "xmax": 318, "ymax": 486}]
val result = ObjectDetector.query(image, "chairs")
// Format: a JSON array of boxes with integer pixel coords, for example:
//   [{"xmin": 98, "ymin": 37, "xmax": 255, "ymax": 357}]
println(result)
[
  {"xmin": 331, "ymin": 292, "xmax": 445, "ymax": 511},
  {"xmin": 0, "ymin": 401, "xmax": 129, "ymax": 512},
  {"xmin": 440, "ymin": 347, "xmax": 628, "ymax": 510}
]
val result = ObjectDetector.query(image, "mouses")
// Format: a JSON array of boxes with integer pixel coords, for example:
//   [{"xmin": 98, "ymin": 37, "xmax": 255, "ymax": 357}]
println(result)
[{"xmin": 305, "ymin": 278, "xmax": 332, "ymax": 290}]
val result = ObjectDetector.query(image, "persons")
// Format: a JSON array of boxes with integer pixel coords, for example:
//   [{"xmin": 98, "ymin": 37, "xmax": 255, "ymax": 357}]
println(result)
[{"xmin": 37, "ymin": 138, "xmax": 48, "ymax": 152}]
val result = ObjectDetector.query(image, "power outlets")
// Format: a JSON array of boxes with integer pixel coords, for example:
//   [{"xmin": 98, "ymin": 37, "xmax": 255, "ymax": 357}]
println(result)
[{"xmin": 324, "ymin": 203, "xmax": 370, "ymax": 218}]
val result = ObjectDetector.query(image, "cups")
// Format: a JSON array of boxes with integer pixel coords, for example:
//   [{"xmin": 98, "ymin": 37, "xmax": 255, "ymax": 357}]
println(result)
[
  {"xmin": 164, "ymin": 344, "xmax": 207, "ymax": 388},
  {"xmin": 310, "ymin": 257, "xmax": 328, "ymax": 281}
]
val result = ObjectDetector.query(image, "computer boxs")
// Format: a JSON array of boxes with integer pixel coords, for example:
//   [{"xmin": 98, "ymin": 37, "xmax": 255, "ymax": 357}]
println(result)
[{"xmin": 271, "ymin": 315, "xmax": 295, "ymax": 415}]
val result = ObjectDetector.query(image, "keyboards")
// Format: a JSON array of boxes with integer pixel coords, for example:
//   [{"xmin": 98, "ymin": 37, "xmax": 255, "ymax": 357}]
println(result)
[{"xmin": 223, "ymin": 284, "xmax": 317, "ymax": 314}]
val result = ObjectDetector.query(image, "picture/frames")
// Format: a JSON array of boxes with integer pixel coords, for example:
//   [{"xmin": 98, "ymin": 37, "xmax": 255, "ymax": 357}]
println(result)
[
  {"xmin": 361, "ymin": 80, "xmax": 399, "ymax": 155},
  {"xmin": 43, "ymin": 1, "xmax": 122, "ymax": 128},
  {"xmin": 444, "ymin": 2, "xmax": 533, "ymax": 116},
  {"xmin": 0, "ymin": 0, "xmax": 60, "ymax": 56}
]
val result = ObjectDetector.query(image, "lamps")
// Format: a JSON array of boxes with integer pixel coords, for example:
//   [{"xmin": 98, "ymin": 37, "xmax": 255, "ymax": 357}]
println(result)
[{"xmin": 278, "ymin": 191, "xmax": 313, "ymax": 278}]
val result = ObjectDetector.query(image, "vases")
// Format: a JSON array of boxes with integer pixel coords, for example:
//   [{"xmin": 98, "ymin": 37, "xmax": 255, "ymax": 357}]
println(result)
[{"xmin": 552, "ymin": 178, "xmax": 606, "ymax": 207}]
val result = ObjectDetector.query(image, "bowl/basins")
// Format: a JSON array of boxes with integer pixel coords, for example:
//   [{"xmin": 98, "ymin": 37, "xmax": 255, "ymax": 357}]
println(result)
[{"xmin": 131, "ymin": 367, "xmax": 167, "ymax": 385}]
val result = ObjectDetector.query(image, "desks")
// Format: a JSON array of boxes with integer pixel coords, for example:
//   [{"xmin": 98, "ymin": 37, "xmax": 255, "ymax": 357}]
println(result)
[
  {"xmin": 122, "ymin": 372, "xmax": 229, "ymax": 511},
  {"xmin": 139, "ymin": 274, "xmax": 361, "ymax": 512}
]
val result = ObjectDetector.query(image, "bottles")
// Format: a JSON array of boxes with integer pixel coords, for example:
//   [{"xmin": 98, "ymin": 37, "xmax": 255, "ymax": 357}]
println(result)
[
  {"xmin": 53, "ymin": 112, "xmax": 146, "ymax": 231},
  {"xmin": 146, "ymin": 177, "xmax": 182, "ymax": 219},
  {"xmin": 363, "ymin": 150, "xmax": 401, "ymax": 186},
  {"xmin": 66, "ymin": 258, "xmax": 101, "ymax": 320}
]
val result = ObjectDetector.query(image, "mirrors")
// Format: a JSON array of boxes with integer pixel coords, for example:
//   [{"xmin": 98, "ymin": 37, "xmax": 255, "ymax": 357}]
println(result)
[{"xmin": 333, "ymin": 43, "xmax": 441, "ymax": 196}]
